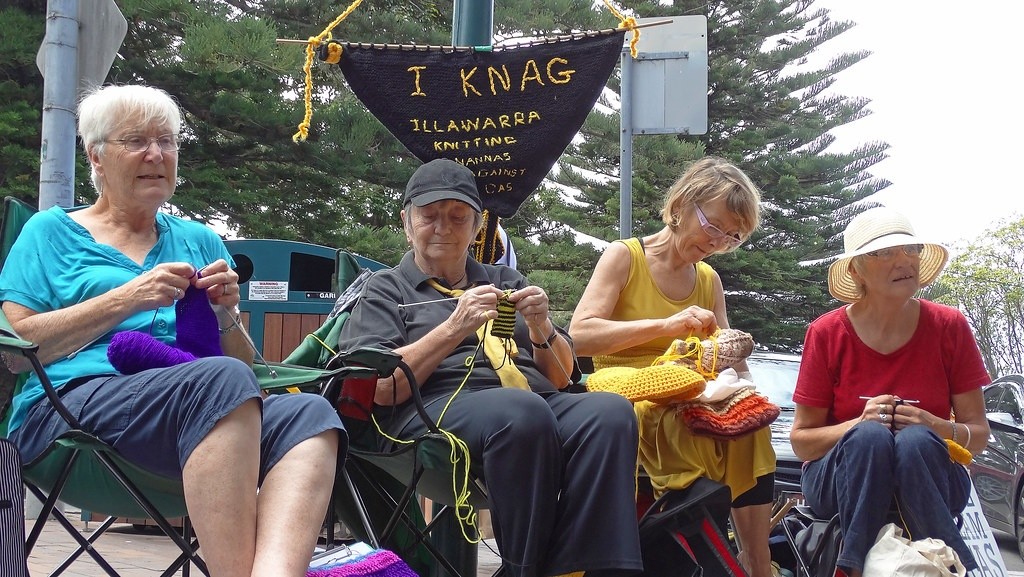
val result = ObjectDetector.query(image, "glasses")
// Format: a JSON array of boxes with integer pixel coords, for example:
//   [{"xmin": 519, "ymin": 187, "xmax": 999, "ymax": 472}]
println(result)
[
  {"xmin": 99, "ymin": 133, "xmax": 183, "ymax": 153},
  {"xmin": 866, "ymin": 244, "xmax": 923, "ymax": 261},
  {"xmin": 693, "ymin": 202, "xmax": 743, "ymax": 248}
]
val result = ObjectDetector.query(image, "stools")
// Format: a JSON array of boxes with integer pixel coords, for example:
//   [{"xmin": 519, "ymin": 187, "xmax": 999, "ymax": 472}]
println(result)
[{"xmin": 781, "ymin": 506, "xmax": 963, "ymax": 577}]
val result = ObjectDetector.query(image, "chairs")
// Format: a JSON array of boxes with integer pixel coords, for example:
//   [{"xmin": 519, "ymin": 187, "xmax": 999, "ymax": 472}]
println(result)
[
  {"xmin": 327, "ymin": 251, "xmax": 509, "ymax": 577},
  {"xmin": 562, "ymin": 326, "xmax": 742, "ymax": 577},
  {"xmin": 0, "ymin": 194, "xmax": 379, "ymax": 577}
]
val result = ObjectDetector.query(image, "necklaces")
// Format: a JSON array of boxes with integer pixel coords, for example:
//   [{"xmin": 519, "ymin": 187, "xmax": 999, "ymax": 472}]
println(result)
[{"xmin": 451, "ymin": 272, "xmax": 466, "ymax": 286}]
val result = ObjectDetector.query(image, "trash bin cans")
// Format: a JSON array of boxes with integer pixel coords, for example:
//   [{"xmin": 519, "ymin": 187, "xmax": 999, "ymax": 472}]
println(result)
[{"xmin": 222, "ymin": 239, "xmax": 394, "ymax": 400}]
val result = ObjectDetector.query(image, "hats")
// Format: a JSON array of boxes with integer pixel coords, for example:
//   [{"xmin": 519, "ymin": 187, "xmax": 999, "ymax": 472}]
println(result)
[
  {"xmin": 403, "ymin": 158, "xmax": 482, "ymax": 213},
  {"xmin": 828, "ymin": 207, "xmax": 949, "ymax": 303}
]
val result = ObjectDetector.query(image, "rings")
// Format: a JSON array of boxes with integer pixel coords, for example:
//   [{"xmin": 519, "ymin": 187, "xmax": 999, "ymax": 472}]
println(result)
[
  {"xmin": 173, "ymin": 287, "xmax": 181, "ymax": 299},
  {"xmin": 880, "ymin": 414, "xmax": 887, "ymax": 422},
  {"xmin": 534, "ymin": 313, "xmax": 538, "ymax": 321},
  {"xmin": 223, "ymin": 283, "xmax": 229, "ymax": 295},
  {"xmin": 879, "ymin": 404, "xmax": 887, "ymax": 413}
]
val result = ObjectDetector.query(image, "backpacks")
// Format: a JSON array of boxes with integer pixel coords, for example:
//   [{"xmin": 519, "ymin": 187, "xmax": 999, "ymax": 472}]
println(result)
[{"xmin": 639, "ymin": 477, "xmax": 750, "ymax": 577}]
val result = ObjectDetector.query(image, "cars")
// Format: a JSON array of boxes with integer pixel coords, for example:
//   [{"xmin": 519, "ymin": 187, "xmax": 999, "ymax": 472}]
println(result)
[
  {"xmin": 971, "ymin": 375, "xmax": 1024, "ymax": 564},
  {"xmin": 745, "ymin": 352, "xmax": 802, "ymax": 503}
]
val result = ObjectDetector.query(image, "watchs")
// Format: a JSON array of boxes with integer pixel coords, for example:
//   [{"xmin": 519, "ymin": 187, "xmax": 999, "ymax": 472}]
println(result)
[{"xmin": 219, "ymin": 306, "xmax": 242, "ymax": 334}]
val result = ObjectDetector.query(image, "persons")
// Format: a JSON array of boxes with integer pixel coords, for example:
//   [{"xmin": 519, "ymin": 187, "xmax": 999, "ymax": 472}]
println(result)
[
  {"xmin": 340, "ymin": 159, "xmax": 643, "ymax": 577},
  {"xmin": 789, "ymin": 207, "xmax": 992, "ymax": 577},
  {"xmin": 568, "ymin": 157, "xmax": 778, "ymax": 577},
  {"xmin": 0, "ymin": 82, "xmax": 348, "ymax": 577}
]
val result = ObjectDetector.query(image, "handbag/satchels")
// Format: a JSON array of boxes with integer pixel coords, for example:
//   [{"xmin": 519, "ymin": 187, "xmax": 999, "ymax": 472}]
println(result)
[
  {"xmin": 863, "ymin": 523, "xmax": 966, "ymax": 577},
  {"xmin": 670, "ymin": 386, "xmax": 780, "ymax": 440},
  {"xmin": 304, "ymin": 544, "xmax": 419, "ymax": 577}
]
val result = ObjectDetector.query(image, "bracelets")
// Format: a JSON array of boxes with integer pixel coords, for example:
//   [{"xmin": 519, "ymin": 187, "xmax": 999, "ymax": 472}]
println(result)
[
  {"xmin": 529, "ymin": 326, "xmax": 557, "ymax": 350},
  {"xmin": 962, "ymin": 422, "xmax": 971, "ymax": 448},
  {"xmin": 948, "ymin": 420, "xmax": 960, "ymax": 442}
]
